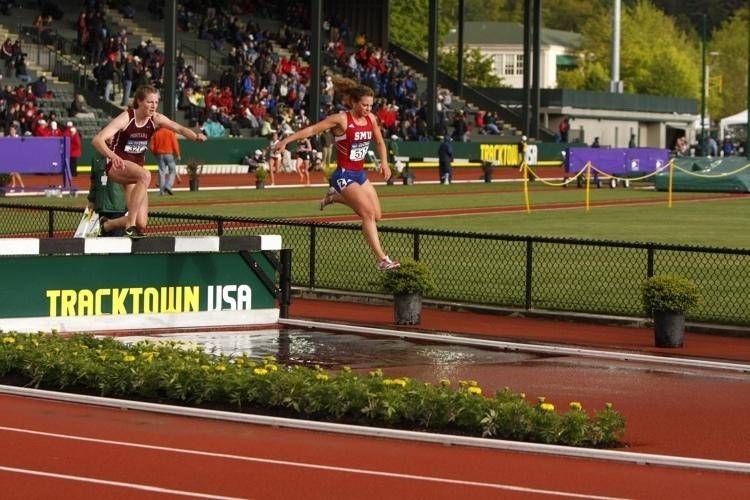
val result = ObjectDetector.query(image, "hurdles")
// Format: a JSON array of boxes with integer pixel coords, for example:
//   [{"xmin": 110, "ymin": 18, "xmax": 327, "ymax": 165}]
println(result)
[{"xmin": 0, "ymin": 235, "xmax": 293, "ymax": 336}]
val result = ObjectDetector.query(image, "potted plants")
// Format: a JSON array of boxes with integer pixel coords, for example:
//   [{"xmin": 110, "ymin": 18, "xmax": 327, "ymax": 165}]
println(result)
[
  {"xmin": 639, "ymin": 273, "xmax": 701, "ymax": 349},
  {"xmin": 370, "ymin": 256, "xmax": 439, "ymax": 325},
  {"xmin": 185, "ymin": 160, "xmax": 205, "ymax": 191},
  {"xmin": 254, "ymin": 166, "xmax": 269, "ymax": 189}
]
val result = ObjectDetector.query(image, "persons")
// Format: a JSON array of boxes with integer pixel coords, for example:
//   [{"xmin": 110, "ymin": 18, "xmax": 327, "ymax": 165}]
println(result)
[
  {"xmin": 91, "ymin": 85, "xmax": 208, "ymax": 239},
  {"xmin": 87, "ymin": 126, "xmax": 129, "ymax": 237},
  {"xmin": 675, "ymin": 132, "xmax": 748, "ymax": 157},
  {"xmin": 629, "ymin": 134, "xmax": 636, "ymax": 148},
  {"xmin": 273, "ymin": 85, "xmax": 401, "ymax": 271},
  {"xmin": 591, "ymin": 137, "xmax": 600, "ymax": 147},
  {"xmin": 559, "ymin": 117, "xmax": 570, "ymax": 143}
]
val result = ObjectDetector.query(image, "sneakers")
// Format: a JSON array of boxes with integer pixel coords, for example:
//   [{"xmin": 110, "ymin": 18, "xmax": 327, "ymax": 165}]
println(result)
[
  {"xmin": 317, "ymin": 184, "xmax": 338, "ymax": 212},
  {"xmin": 96, "ymin": 216, "xmax": 110, "ymax": 238},
  {"xmin": 374, "ymin": 254, "xmax": 402, "ymax": 271},
  {"xmin": 121, "ymin": 226, "xmax": 147, "ymax": 240},
  {"xmin": 160, "ymin": 186, "xmax": 174, "ymax": 197}
]
